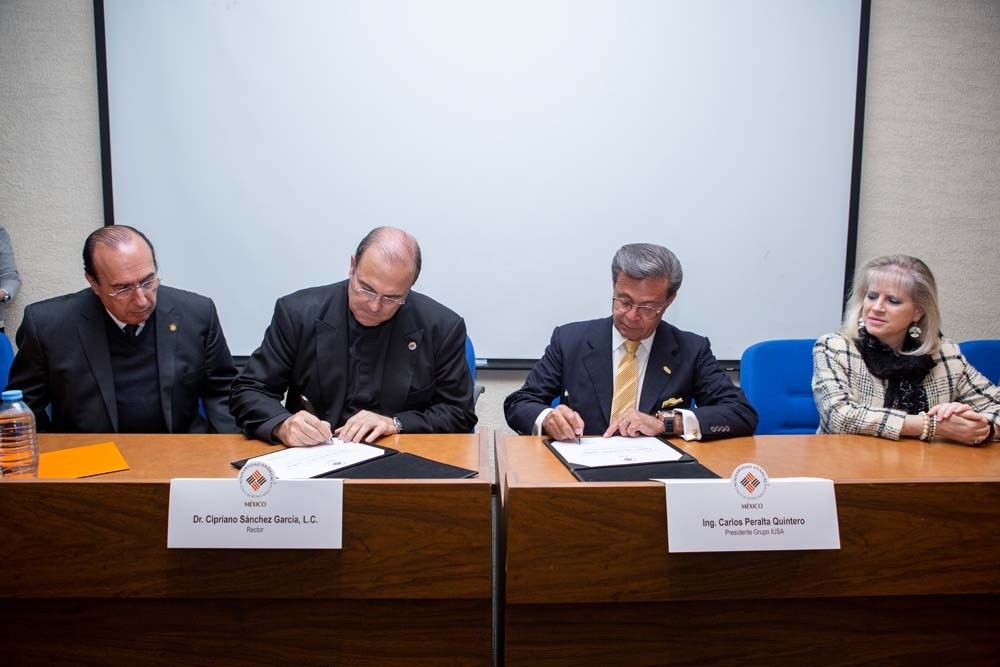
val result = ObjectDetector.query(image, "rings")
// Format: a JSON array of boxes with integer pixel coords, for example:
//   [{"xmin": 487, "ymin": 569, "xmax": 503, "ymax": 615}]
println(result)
[{"xmin": 974, "ymin": 440, "xmax": 977, "ymax": 444}]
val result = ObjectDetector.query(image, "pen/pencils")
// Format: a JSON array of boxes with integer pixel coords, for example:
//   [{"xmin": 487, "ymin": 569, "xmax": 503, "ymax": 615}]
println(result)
[
  {"xmin": 300, "ymin": 394, "xmax": 334, "ymax": 443},
  {"xmin": 564, "ymin": 389, "xmax": 580, "ymax": 444}
]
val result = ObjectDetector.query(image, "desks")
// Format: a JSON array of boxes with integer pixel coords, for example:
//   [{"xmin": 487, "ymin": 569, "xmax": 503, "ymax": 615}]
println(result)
[{"xmin": 0, "ymin": 434, "xmax": 1000, "ymax": 667}]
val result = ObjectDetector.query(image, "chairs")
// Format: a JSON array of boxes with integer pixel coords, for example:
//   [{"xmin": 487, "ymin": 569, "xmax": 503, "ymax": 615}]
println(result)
[
  {"xmin": 739, "ymin": 338, "xmax": 820, "ymax": 435},
  {"xmin": 958, "ymin": 340, "xmax": 1000, "ymax": 387}
]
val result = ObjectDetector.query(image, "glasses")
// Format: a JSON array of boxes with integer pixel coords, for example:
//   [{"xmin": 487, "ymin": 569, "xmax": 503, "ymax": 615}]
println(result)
[
  {"xmin": 98, "ymin": 277, "xmax": 162, "ymax": 302},
  {"xmin": 353, "ymin": 276, "xmax": 406, "ymax": 308},
  {"xmin": 611, "ymin": 298, "xmax": 666, "ymax": 320}
]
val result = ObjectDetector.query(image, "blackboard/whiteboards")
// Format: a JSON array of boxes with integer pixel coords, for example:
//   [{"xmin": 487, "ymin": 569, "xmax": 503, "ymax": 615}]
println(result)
[{"xmin": 91, "ymin": 0, "xmax": 871, "ymax": 371}]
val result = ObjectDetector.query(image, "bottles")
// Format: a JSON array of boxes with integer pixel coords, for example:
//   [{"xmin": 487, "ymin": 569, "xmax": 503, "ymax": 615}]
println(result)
[{"xmin": 0, "ymin": 390, "xmax": 39, "ymax": 480}]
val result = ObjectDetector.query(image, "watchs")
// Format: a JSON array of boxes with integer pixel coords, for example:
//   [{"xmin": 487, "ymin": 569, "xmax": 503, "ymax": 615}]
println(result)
[
  {"xmin": 393, "ymin": 416, "xmax": 403, "ymax": 433},
  {"xmin": 656, "ymin": 410, "xmax": 675, "ymax": 436}
]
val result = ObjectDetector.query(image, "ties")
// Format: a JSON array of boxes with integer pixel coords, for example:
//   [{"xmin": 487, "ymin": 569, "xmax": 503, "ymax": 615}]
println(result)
[{"xmin": 611, "ymin": 340, "xmax": 642, "ymax": 424}]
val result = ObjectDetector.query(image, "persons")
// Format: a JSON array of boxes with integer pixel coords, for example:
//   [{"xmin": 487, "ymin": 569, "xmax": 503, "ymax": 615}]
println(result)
[
  {"xmin": 229, "ymin": 227, "xmax": 479, "ymax": 447},
  {"xmin": 503, "ymin": 242, "xmax": 758, "ymax": 442},
  {"xmin": 5, "ymin": 226, "xmax": 246, "ymax": 435},
  {"xmin": 810, "ymin": 255, "xmax": 1000, "ymax": 446},
  {"xmin": 0, "ymin": 226, "xmax": 21, "ymax": 332}
]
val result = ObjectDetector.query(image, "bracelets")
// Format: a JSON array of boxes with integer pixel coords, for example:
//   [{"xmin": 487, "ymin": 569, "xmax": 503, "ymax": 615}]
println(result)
[
  {"xmin": 1, "ymin": 288, "xmax": 9, "ymax": 302},
  {"xmin": 919, "ymin": 412, "xmax": 937, "ymax": 442},
  {"xmin": 988, "ymin": 420, "xmax": 995, "ymax": 442}
]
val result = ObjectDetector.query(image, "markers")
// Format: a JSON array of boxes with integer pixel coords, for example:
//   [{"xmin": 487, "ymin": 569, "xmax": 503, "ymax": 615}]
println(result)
[{"xmin": 476, "ymin": 360, "xmax": 487, "ymax": 366}]
